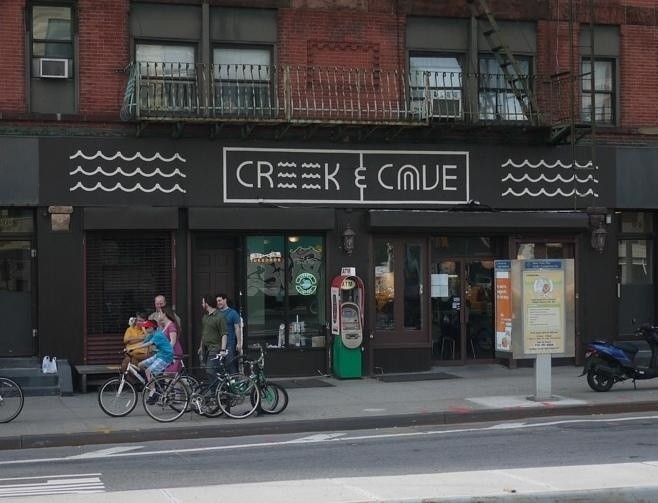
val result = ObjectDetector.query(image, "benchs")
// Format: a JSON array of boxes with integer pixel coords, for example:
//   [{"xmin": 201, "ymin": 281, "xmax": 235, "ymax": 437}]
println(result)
[{"xmin": 75, "ymin": 364, "xmax": 122, "ymax": 395}]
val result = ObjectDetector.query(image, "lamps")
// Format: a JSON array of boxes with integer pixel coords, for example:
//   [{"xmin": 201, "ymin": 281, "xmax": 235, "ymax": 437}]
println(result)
[
  {"xmin": 594, "ymin": 220, "xmax": 608, "ymax": 255},
  {"xmin": 341, "ymin": 223, "xmax": 356, "ymax": 256}
]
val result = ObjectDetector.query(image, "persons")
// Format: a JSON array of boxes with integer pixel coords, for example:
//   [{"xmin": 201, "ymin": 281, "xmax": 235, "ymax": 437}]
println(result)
[
  {"xmin": 121, "ymin": 311, "xmax": 153, "ymax": 374},
  {"xmin": 197, "ymin": 293, "xmax": 229, "ymax": 414},
  {"xmin": 148, "ymin": 294, "xmax": 181, "ymax": 329},
  {"xmin": 159, "ymin": 305, "xmax": 183, "ymax": 390},
  {"xmin": 126, "ymin": 320, "xmax": 174, "ymax": 405},
  {"xmin": 216, "ymin": 294, "xmax": 241, "ymax": 384}
]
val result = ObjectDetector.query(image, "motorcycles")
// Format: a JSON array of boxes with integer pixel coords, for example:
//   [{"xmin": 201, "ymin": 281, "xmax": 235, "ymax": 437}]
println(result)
[{"xmin": 576, "ymin": 316, "xmax": 657, "ymax": 393}]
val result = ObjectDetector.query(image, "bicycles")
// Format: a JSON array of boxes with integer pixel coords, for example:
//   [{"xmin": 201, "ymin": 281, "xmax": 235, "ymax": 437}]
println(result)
[
  {"xmin": 0, "ymin": 376, "xmax": 24, "ymax": 422},
  {"xmin": 97, "ymin": 347, "xmax": 288, "ymax": 422}
]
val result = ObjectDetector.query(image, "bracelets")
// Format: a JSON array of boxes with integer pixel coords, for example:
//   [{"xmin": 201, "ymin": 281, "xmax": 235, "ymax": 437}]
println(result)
[{"xmin": 220, "ymin": 349, "xmax": 226, "ymax": 352}]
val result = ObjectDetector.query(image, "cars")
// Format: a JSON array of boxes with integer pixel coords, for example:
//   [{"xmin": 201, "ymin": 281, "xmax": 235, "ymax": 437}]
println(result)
[{"xmin": 247, "ymin": 296, "xmax": 317, "ymax": 316}]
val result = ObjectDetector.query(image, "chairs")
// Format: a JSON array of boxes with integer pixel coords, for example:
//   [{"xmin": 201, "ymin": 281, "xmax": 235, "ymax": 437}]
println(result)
[{"xmin": 440, "ymin": 322, "xmax": 476, "ymax": 361}]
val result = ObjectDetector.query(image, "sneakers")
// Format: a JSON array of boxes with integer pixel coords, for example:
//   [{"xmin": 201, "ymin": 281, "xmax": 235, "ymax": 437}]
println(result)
[{"xmin": 145, "ymin": 390, "xmax": 161, "ymax": 405}]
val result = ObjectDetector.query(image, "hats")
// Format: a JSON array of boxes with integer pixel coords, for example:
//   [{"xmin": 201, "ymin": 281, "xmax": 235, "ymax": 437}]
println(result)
[{"xmin": 142, "ymin": 321, "xmax": 157, "ymax": 328}]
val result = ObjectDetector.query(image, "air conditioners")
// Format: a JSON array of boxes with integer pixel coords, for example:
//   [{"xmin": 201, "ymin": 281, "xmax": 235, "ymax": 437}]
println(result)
[
  {"xmin": 424, "ymin": 98, "xmax": 462, "ymax": 119},
  {"xmin": 39, "ymin": 57, "xmax": 69, "ymax": 79}
]
